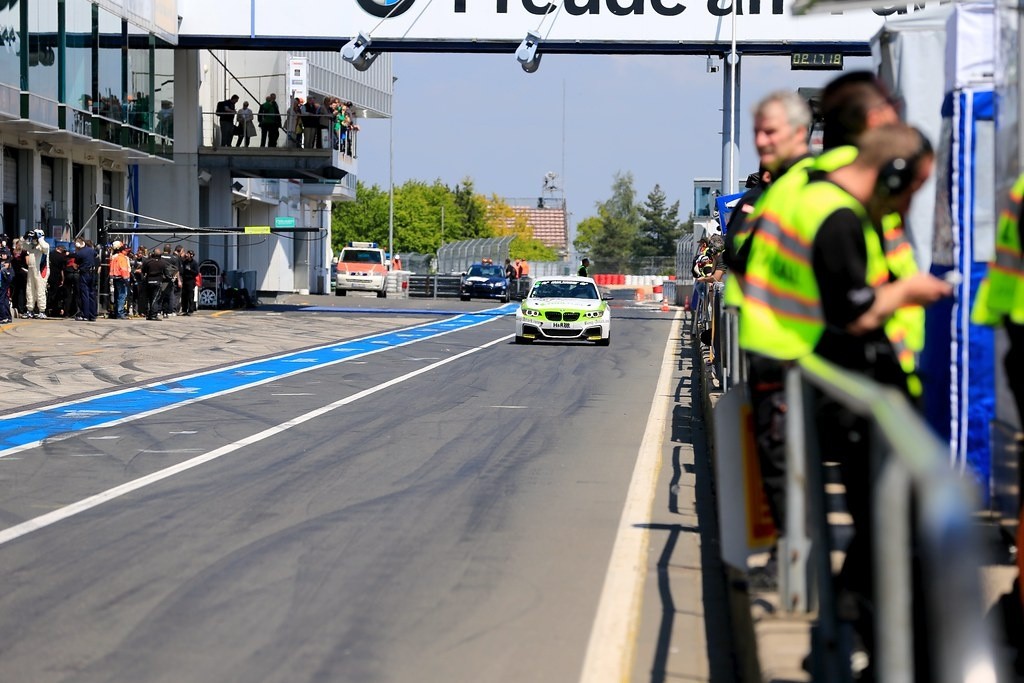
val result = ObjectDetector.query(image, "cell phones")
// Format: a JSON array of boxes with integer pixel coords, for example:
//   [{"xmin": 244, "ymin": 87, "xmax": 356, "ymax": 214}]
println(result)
[{"xmin": 940, "ymin": 271, "xmax": 963, "ymax": 287}]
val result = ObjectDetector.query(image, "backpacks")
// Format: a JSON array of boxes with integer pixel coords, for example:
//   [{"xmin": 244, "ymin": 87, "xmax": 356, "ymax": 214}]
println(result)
[{"xmin": 509, "ymin": 265, "xmax": 517, "ymax": 278}]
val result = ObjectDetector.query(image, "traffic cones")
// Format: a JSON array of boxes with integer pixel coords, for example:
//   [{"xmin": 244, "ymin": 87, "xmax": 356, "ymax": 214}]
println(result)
[
  {"xmin": 661, "ymin": 296, "xmax": 670, "ymax": 310},
  {"xmin": 685, "ymin": 295, "xmax": 689, "ymax": 310}
]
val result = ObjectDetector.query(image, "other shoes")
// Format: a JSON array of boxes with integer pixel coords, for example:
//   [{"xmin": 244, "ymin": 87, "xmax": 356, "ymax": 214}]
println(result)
[
  {"xmin": 89, "ymin": 317, "xmax": 96, "ymax": 321},
  {"xmin": 78, "ymin": 316, "xmax": 88, "ymax": 321},
  {"xmin": 164, "ymin": 312, "xmax": 176, "ymax": 318},
  {"xmin": 177, "ymin": 311, "xmax": 187, "ymax": 316},
  {"xmin": 712, "ymin": 385, "xmax": 723, "ymax": 390},
  {"xmin": 187, "ymin": 312, "xmax": 192, "ymax": 315},
  {"xmin": 147, "ymin": 318, "xmax": 162, "ymax": 321},
  {"xmin": 22, "ymin": 311, "xmax": 34, "ymax": 318},
  {"xmin": 707, "ymin": 362, "xmax": 711, "ymax": 366},
  {"xmin": 737, "ymin": 559, "xmax": 778, "ymax": 592},
  {"xmin": 36, "ymin": 312, "xmax": 48, "ymax": 319}
]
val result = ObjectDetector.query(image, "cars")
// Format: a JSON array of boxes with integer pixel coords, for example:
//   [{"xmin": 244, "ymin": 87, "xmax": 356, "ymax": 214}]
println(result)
[
  {"xmin": 515, "ymin": 275, "xmax": 614, "ymax": 346},
  {"xmin": 459, "ymin": 259, "xmax": 510, "ymax": 302},
  {"xmin": 331, "ymin": 242, "xmax": 389, "ymax": 297}
]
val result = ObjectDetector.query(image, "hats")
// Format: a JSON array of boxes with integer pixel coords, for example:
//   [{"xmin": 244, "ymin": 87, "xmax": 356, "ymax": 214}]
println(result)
[
  {"xmin": 516, "ymin": 259, "xmax": 520, "ymax": 263},
  {"xmin": 120, "ymin": 244, "xmax": 132, "ymax": 252}
]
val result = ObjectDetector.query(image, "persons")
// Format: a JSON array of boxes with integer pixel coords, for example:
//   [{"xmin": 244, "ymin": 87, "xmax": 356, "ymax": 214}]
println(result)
[
  {"xmin": 283, "ymin": 89, "xmax": 357, "ymax": 157},
  {"xmin": 72, "ymin": 89, "xmax": 174, "ymax": 146},
  {"xmin": 481, "ymin": 257, "xmax": 493, "ymax": 266},
  {"xmin": 504, "ymin": 257, "xmax": 529, "ymax": 295},
  {"xmin": 577, "ymin": 258, "xmax": 590, "ymax": 277},
  {"xmin": 219, "ymin": 94, "xmax": 239, "ymax": 147},
  {"xmin": 258, "ymin": 93, "xmax": 282, "ymax": 147},
  {"xmin": 392, "ymin": 255, "xmax": 402, "ymax": 270},
  {"xmin": 691, "ymin": 73, "xmax": 1024, "ymax": 683},
  {"xmin": 236, "ymin": 101, "xmax": 257, "ymax": 147},
  {"xmin": 0, "ymin": 229, "xmax": 200, "ymax": 324}
]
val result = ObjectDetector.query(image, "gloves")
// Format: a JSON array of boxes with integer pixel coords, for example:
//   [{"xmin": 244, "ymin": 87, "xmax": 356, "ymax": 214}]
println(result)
[
  {"xmin": 23, "ymin": 230, "xmax": 30, "ymax": 240},
  {"xmin": 33, "ymin": 232, "xmax": 42, "ymax": 239}
]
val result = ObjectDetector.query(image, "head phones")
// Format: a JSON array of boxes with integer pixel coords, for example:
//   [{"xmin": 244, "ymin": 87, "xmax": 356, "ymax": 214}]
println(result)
[{"xmin": 873, "ymin": 130, "xmax": 932, "ymax": 198}]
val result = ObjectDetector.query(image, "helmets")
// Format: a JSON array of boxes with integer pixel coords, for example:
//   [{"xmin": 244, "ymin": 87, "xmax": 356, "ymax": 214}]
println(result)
[{"xmin": 395, "ymin": 255, "xmax": 400, "ymax": 259}]
